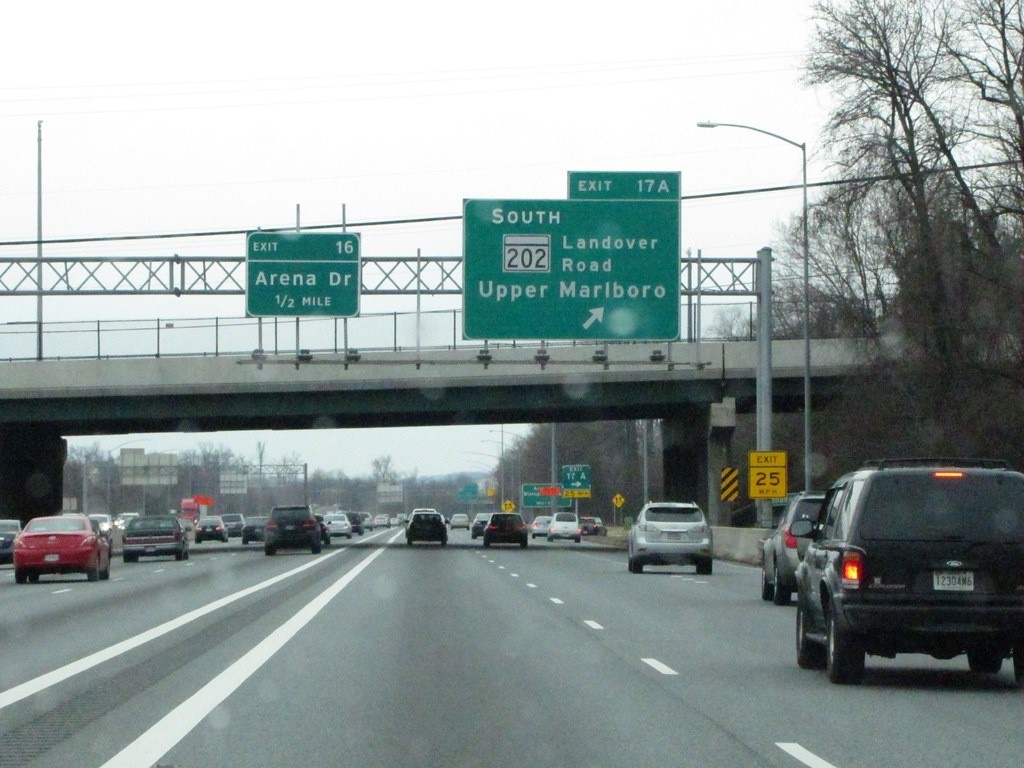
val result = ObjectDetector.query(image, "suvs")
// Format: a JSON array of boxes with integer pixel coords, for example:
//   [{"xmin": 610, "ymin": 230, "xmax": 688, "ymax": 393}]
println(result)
[{"xmin": 788, "ymin": 453, "xmax": 1024, "ymax": 685}]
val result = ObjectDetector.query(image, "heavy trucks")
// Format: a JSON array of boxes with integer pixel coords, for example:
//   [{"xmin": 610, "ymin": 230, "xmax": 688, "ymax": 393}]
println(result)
[{"xmin": 179, "ymin": 498, "xmax": 208, "ymax": 525}]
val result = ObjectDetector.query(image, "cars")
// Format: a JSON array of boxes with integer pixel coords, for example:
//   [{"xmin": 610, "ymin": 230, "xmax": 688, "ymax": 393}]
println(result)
[
  {"xmin": 14, "ymin": 515, "xmax": 112, "ymax": 583},
  {"xmin": 83, "ymin": 513, "xmax": 139, "ymax": 533},
  {"xmin": 471, "ymin": 513, "xmax": 494, "ymax": 540},
  {"xmin": 122, "ymin": 515, "xmax": 193, "ymax": 561},
  {"xmin": 451, "ymin": 514, "xmax": 470, "ymax": 531},
  {"xmin": 627, "ymin": 500, "xmax": 714, "ymax": 574},
  {"xmin": 531, "ymin": 516, "xmax": 553, "ymax": 539},
  {"xmin": 483, "ymin": 512, "xmax": 528, "ymax": 548},
  {"xmin": 400, "ymin": 508, "xmax": 451, "ymax": 545},
  {"xmin": 578, "ymin": 516, "xmax": 608, "ymax": 536},
  {"xmin": 0, "ymin": 519, "xmax": 24, "ymax": 564},
  {"xmin": 546, "ymin": 512, "xmax": 581, "ymax": 543},
  {"xmin": 194, "ymin": 504, "xmax": 404, "ymax": 555},
  {"xmin": 758, "ymin": 493, "xmax": 823, "ymax": 606}
]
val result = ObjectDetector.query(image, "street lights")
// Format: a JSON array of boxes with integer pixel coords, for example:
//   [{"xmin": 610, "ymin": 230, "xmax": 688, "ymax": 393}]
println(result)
[
  {"xmin": 471, "ymin": 429, "xmax": 528, "ymax": 511},
  {"xmin": 696, "ymin": 121, "xmax": 813, "ymax": 495}
]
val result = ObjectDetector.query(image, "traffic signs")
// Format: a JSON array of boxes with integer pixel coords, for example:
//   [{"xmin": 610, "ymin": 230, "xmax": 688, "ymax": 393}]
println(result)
[
  {"xmin": 462, "ymin": 199, "xmax": 682, "ymax": 340},
  {"xmin": 561, "ymin": 464, "xmax": 591, "ymax": 491}
]
